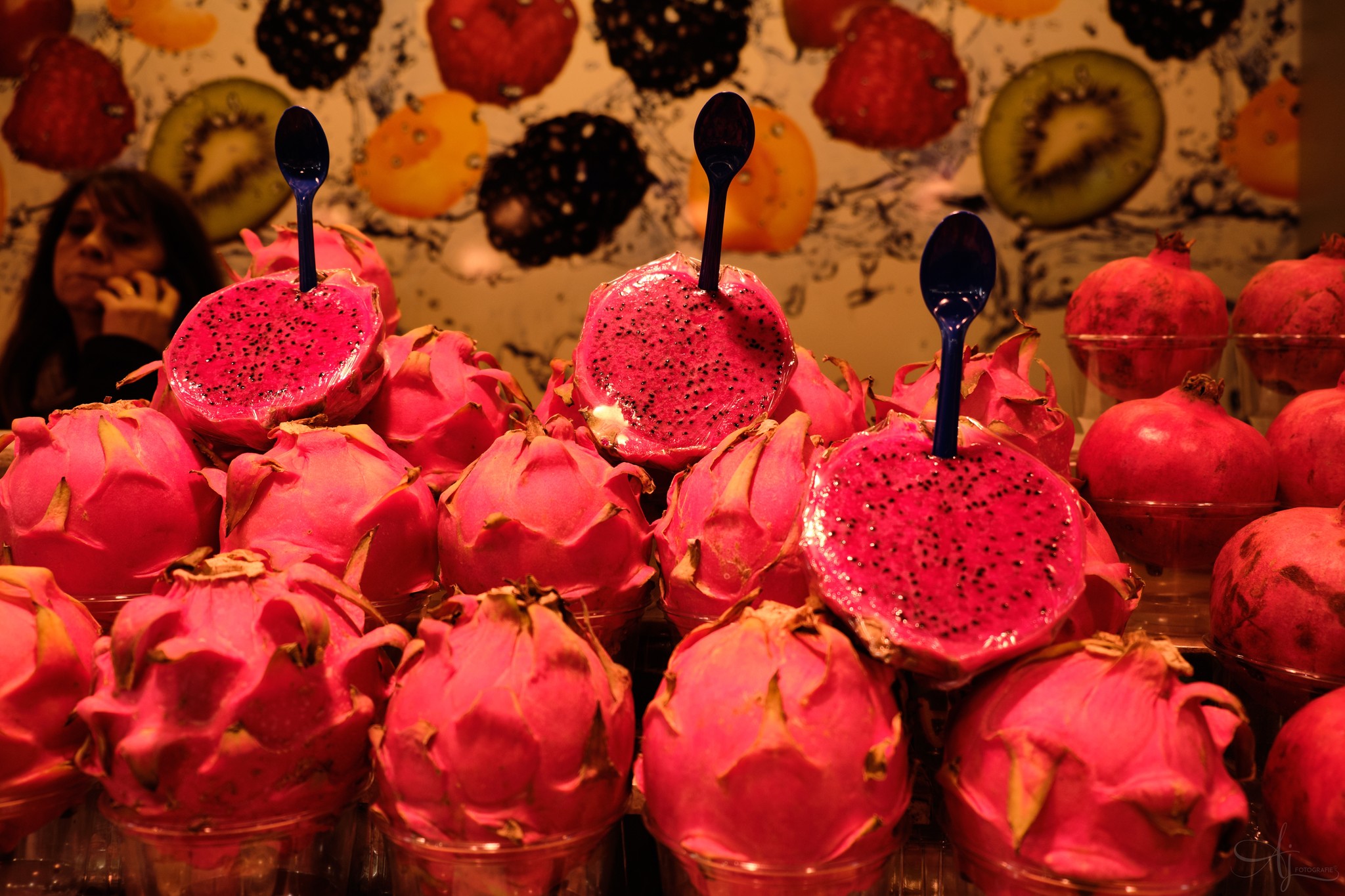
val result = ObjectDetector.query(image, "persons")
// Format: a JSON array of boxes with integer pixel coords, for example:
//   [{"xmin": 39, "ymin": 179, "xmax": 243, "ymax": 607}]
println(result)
[{"xmin": 0, "ymin": 164, "xmax": 226, "ymax": 421}]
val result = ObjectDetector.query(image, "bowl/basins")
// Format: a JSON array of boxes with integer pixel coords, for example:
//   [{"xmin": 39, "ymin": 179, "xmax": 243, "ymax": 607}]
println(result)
[{"xmin": 2, "ymin": 331, "xmax": 1331, "ymax": 896}]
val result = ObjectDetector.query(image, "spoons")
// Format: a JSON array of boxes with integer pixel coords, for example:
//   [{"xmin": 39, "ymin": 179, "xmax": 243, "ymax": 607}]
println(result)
[
  {"xmin": 919, "ymin": 210, "xmax": 999, "ymax": 458},
  {"xmin": 692, "ymin": 90, "xmax": 757, "ymax": 293},
  {"xmin": 275, "ymin": 105, "xmax": 330, "ymax": 293}
]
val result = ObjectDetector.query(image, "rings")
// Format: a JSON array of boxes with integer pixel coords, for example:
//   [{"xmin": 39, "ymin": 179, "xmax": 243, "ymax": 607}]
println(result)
[{"xmin": 118, "ymin": 288, "xmax": 134, "ymax": 298}]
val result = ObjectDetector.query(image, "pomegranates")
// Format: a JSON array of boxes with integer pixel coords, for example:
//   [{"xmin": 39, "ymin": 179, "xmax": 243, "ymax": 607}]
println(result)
[
  {"xmin": 1260, "ymin": 686, "xmax": 1345, "ymax": 884},
  {"xmin": 1078, "ymin": 369, "xmax": 1278, "ymax": 571},
  {"xmin": 1208, "ymin": 498, "xmax": 1345, "ymax": 683},
  {"xmin": 1264, "ymin": 369, "xmax": 1345, "ymax": 508},
  {"xmin": 1229, "ymin": 227, "xmax": 1345, "ymax": 398},
  {"xmin": 1065, "ymin": 231, "xmax": 1229, "ymax": 401}
]
